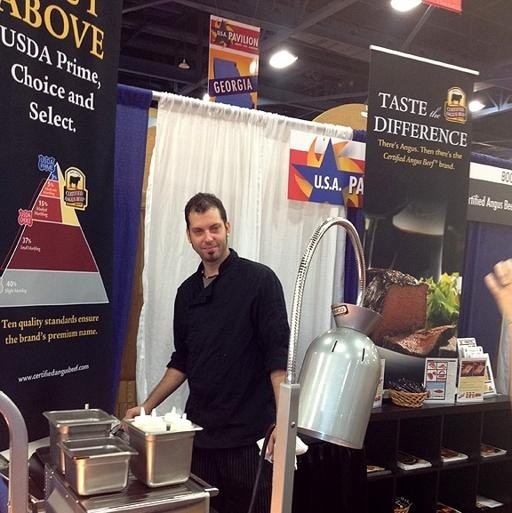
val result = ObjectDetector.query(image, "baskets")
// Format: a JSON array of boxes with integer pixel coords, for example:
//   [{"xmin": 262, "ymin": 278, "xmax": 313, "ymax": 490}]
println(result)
[{"xmin": 389, "ymin": 388, "xmax": 428, "ymax": 407}]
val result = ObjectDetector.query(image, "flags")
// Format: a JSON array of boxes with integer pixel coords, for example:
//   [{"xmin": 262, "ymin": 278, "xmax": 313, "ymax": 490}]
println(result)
[
  {"xmin": 208, "ymin": 12, "xmax": 263, "ymax": 108},
  {"xmin": 363, "ymin": 43, "xmax": 477, "ymax": 225},
  {"xmin": 286, "ymin": 127, "xmax": 365, "ymax": 208}
]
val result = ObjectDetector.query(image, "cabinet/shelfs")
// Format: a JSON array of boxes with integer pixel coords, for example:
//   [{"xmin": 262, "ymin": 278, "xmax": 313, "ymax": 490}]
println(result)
[{"xmin": 365, "ymin": 388, "xmax": 511, "ymax": 511}]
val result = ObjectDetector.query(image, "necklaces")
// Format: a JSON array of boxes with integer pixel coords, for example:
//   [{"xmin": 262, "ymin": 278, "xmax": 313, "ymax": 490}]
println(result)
[{"xmin": 201, "ymin": 270, "xmax": 219, "ymax": 279}]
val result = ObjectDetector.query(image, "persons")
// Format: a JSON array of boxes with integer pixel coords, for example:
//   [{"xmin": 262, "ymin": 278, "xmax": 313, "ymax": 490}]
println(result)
[
  {"xmin": 125, "ymin": 190, "xmax": 294, "ymax": 513},
  {"xmin": 484, "ymin": 259, "xmax": 512, "ymax": 398}
]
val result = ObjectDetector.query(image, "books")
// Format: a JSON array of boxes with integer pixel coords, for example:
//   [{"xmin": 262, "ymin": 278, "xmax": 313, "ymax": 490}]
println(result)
[
  {"xmin": 364, "ymin": 442, "xmax": 508, "ymax": 512},
  {"xmin": 422, "ymin": 335, "xmax": 497, "ymax": 406}
]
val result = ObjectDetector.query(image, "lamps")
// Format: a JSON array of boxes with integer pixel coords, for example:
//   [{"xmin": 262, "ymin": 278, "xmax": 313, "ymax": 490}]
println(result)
[{"xmin": 271, "ymin": 213, "xmax": 381, "ymax": 512}]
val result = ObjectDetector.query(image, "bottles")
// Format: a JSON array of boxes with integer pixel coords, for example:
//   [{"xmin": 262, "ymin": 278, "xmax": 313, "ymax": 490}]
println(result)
[{"xmin": 135, "ymin": 405, "xmax": 191, "ymax": 433}]
